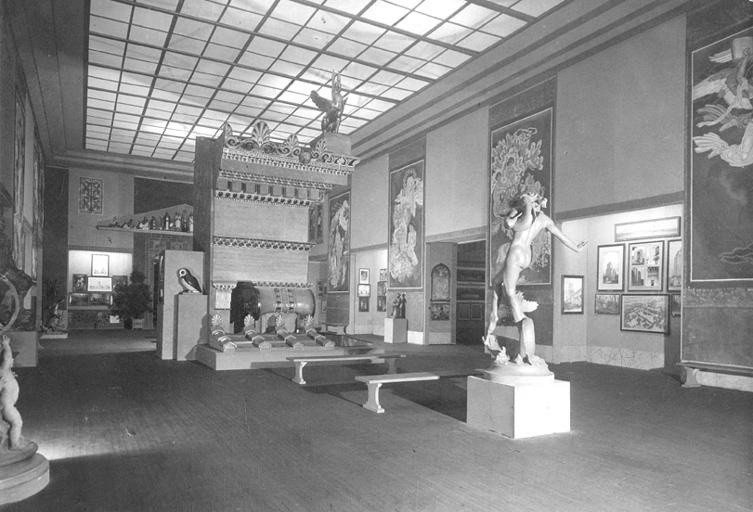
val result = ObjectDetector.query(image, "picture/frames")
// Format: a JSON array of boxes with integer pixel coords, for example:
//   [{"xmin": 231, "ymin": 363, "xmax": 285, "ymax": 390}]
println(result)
[
  {"xmin": 560, "ymin": 214, "xmax": 684, "ymax": 337},
  {"xmin": 355, "ymin": 267, "xmax": 388, "ymax": 312},
  {"xmin": 429, "ymin": 260, "xmax": 486, "ymax": 323},
  {"xmin": 66, "ymin": 251, "xmax": 128, "ymax": 306}
]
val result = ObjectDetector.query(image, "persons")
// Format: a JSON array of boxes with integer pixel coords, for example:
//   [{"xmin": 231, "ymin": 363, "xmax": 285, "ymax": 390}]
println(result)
[
  {"xmin": 0, "ymin": 336, "xmax": 23, "ymax": 451},
  {"xmin": 486, "ymin": 189, "xmax": 590, "ymax": 360},
  {"xmin": 390, "ymin": 168, "xmax": 424, "ymax": 279}
]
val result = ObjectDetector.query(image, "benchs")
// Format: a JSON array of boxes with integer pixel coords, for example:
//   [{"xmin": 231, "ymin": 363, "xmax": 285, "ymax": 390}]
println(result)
[
  {"xmin": 284, "ymin": 353, "xmax": 408, "ymax": 385},
  {"xmin": 352, "ymin": 369, "xmax": 485, "ymax": 414}
]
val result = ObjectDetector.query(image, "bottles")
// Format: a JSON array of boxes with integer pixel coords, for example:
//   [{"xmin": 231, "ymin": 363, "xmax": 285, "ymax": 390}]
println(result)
[{"xmin": 110, "ymin": 210, "xmax": 192, "ymax": 233}]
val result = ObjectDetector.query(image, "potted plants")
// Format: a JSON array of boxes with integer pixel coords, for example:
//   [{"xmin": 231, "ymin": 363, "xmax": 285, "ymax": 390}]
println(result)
[{"xmin": 111, "ymin": 270, "xmax": 152, "ymax": 329}]
